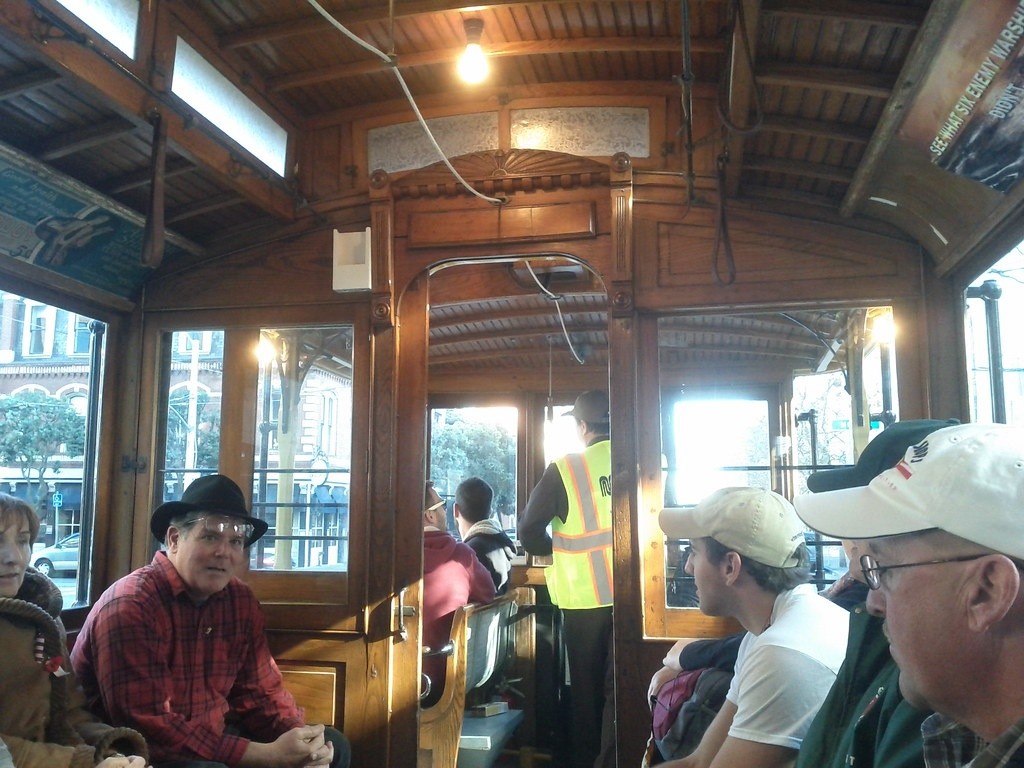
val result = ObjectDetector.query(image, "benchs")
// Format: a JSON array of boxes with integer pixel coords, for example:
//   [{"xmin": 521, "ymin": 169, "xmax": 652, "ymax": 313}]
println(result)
[{"xmin": 417, "ymin": 588, "xmax": 519, "ymax": 768}]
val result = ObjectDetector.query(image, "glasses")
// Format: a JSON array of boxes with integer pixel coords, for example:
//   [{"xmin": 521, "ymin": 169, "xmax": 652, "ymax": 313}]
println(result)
[
  {"xmin": 425, "ymin": 499, "xmax": 447, "ymax": 515},
  {"xmin": 860, "ymin": 554, "xmax": 1024, "ymax": 590},
  {"xmin": 186, "ymin": 513, "xmax": 255, "ymax": 539}
]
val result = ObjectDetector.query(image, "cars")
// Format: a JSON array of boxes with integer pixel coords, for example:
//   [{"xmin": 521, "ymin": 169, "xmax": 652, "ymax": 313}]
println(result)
[
  {"xmin": 27, "ymin": 531, "xmax": 80, "ymax": 579},
  {"xmin": 249, "ymin": 552, "xmax": 296, "ymax": 570}
]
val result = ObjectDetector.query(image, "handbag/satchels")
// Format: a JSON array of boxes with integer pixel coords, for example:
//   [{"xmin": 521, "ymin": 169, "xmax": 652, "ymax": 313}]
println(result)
[{"xmin": 651, "ymin": 668, "xmax": 733, "ymax": 760}]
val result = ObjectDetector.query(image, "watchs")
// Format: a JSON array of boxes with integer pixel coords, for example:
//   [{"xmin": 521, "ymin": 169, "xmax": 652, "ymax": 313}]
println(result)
[{"xmin": 103, "ymin": 751, "xmax": 125, "ymax": 760}]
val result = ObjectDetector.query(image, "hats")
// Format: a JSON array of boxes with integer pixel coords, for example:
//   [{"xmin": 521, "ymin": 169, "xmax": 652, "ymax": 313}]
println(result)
[
  {"xmin": 659, "ymin": 486, "xmax": 804, "ymax": 568},
  {"xmin": 793, "ymin": 422, "xmax": 1024, "ymax": 561},
  {"xmin": 807, "ymin": 418, "xmax": 962, "ymax": 492},
  {"xmin": 562, "ymin": 391, "xmax": 610, "ymax": 424},
  {"xmin": 150, "ymin": 474, "xmax": 268, "ymax": 548}
]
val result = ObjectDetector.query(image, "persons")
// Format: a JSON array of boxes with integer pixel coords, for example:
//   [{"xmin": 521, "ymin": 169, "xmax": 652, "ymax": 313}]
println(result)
[
  {"xmin": 421, "ymin": 477, "xmax": 517, "ymax": 693},
  {"xmin": 647, "ymin": 417, "xmax": 1024, "ymax": 768},
  {"xmin": 69, "ymin": 475, "xmax": 351, "ymax": 768},
  {"xmin": 517, "ymin": 389, "xmax": 614, "ymax": 768},
  {"xmin": 0, "ymin": 492, "xmax": 150, "ymax": 768}
]
